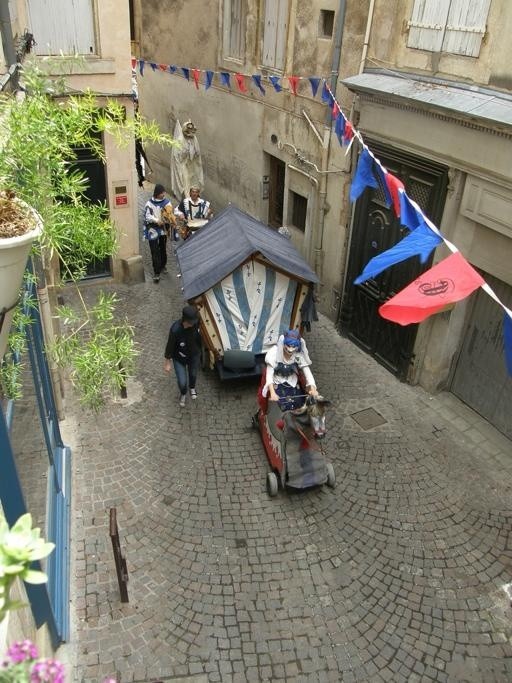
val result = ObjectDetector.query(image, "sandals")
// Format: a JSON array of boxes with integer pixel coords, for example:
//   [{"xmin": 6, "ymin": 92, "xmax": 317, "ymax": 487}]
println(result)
[{"xmin": 178, "ymin": 388, "xmax": 198, "ymax": 408}]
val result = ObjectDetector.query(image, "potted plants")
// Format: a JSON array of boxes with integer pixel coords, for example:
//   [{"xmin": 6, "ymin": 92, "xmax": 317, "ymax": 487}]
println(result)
[{"xmin": 0, "ymin": 46, "xmax": 181, "ymax": 413}]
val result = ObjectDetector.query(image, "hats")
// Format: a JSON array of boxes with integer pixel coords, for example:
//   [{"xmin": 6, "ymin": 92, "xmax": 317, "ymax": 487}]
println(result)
[
  {"xmin": 154, "ymin": 183, "xmax": 165, "ymax": 195},
  {"xmin": 182, "ymin": 305, "xmax": 199, "ymax": 324}
]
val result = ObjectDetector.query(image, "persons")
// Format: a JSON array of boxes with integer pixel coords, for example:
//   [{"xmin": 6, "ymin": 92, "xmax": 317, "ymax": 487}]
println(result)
[
  {"xmin": 174, "ymin": 185, "xmax": 213, "ymax": 239},
  {"xmin": 265, "ymin": 339, "xmax": 317, "ymax": 402},
  {"xmin": 144, "ymin": 184, "xmax": 175, "ymax": 283},
  {"xmin": 277, "ymin": 330, "xmax": 311, "ymax": 365},
  {"xmin": 164, "ymin": 305, "xmax": 201, "ymax": 406}
]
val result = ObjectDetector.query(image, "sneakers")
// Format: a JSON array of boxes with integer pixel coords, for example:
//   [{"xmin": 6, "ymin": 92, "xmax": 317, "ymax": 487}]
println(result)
[{"xmin": 151, "ymin": 267, "xmax": 168, "ymax": 282}]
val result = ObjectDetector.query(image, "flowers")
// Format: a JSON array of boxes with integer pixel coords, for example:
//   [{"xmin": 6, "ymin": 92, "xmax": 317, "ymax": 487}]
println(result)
[{"xmin": 0, "ymin": 512, "xmax": 118, "ymax": 683}]
